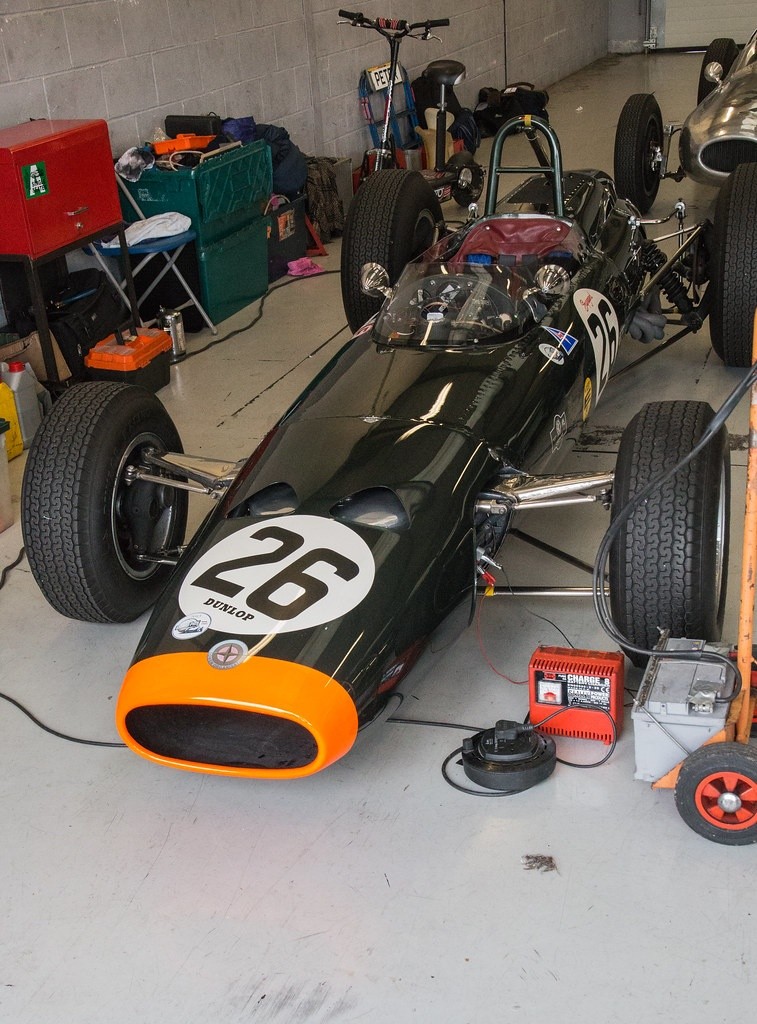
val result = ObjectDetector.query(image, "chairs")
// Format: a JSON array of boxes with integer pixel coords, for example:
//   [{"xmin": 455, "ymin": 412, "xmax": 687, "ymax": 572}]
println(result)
[{"xmin": 80, "ymin": 171, "xmax": 218, "ymax": 336}]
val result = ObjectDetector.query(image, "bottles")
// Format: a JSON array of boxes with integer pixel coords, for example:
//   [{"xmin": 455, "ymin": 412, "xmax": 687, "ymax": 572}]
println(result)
[{"xmin": 0, "ymin": 360, "xmax": 42, "ymax": 534}]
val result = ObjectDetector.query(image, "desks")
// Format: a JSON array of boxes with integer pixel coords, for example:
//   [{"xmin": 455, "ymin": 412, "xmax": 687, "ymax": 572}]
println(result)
[{"xmin": 0, "ymin": 222, "xmax": 140, "ymax": 401}]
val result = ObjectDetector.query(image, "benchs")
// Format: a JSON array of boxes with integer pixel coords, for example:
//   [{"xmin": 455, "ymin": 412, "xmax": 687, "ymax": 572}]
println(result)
[{"xmin": 448, "ymin": 212, "xmax": 587, "ymax": 296}]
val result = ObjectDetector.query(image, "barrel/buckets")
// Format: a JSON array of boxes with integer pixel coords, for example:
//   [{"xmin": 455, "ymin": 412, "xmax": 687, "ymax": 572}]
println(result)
[{"xmin": 390, "ymin": 150, "xmax": 423, "ymax": 171}]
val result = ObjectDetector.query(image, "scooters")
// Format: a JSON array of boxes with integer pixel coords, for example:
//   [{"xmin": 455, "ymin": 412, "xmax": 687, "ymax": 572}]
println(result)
[{"xmin": 334, "ymin": 8, "xmax": 489, "ymax": 222}]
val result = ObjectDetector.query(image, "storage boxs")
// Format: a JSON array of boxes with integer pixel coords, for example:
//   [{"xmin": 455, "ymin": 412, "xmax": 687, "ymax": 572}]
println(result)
[{"xmin": 0, "ymin": 118, "xmax": 354, "ymax": 382}]
